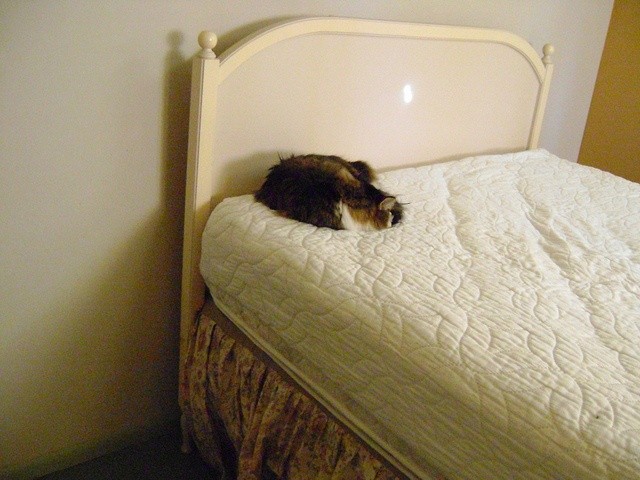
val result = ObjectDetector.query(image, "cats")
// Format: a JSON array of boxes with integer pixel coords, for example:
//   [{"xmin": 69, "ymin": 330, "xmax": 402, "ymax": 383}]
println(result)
[{"xmin": 253, "ymin": 152, "xmax": 410, "ymax": 232}]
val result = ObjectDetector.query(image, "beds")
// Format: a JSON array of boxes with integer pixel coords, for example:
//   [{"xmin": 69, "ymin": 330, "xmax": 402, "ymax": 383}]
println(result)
[{"xmin": 174, "ymin": 13, "xmax": 639, "ymax": 479}]
